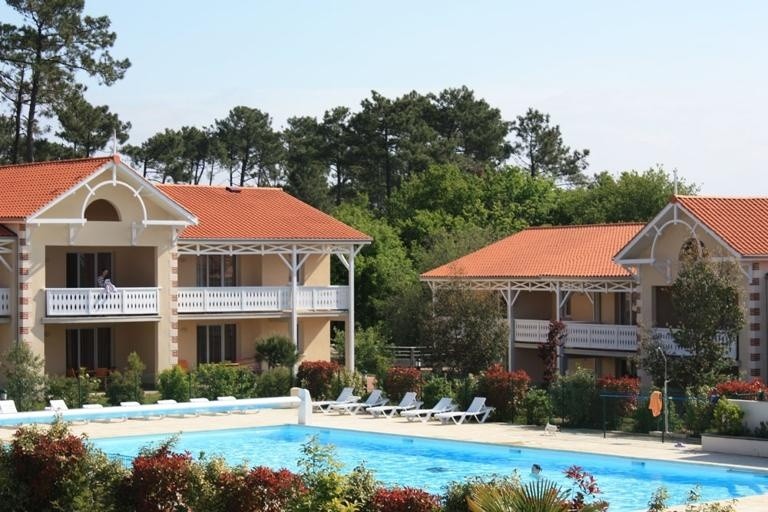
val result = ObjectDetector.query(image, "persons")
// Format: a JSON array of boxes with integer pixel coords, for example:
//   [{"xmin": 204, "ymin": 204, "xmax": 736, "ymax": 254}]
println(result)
[
  {"xmin": 97, "ymin": 267, "xmax": 110, "ymax": 287},
  {"xmin": 107, "ymin": 366, "xmax": 123, "ymax": 386}
]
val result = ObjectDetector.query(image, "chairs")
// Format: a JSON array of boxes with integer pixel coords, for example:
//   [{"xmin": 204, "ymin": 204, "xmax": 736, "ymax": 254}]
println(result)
[
  {"xmin": 95, "ymin": 368, "xmax": 108, "ymax": 376},
  {"xmin": 178, "ymin": 360, "xmax": 191, "ymax": 383},
  {"xmin": 312, "ymin": 387, "xmax": 497, "ymax": 425},
  {"xmin": 0, "ymin": 400, "xmax": 23, "ymax": 426},
  {"xmin": 67, "ymin": 367, "xmax": 75, "ymax": 378},
  {"xmin": 111, "ymin": 367, "xmax": 126, "ymax": 373},
  {"xmin": 45, "ymin": 396, "xmax": 259, "ymax": 426}
]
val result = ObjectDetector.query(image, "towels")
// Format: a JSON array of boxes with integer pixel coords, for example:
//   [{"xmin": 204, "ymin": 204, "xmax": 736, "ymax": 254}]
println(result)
[{"xmin": 648, "ymin": 390, "xmax": 662, "ymax": 417}]
